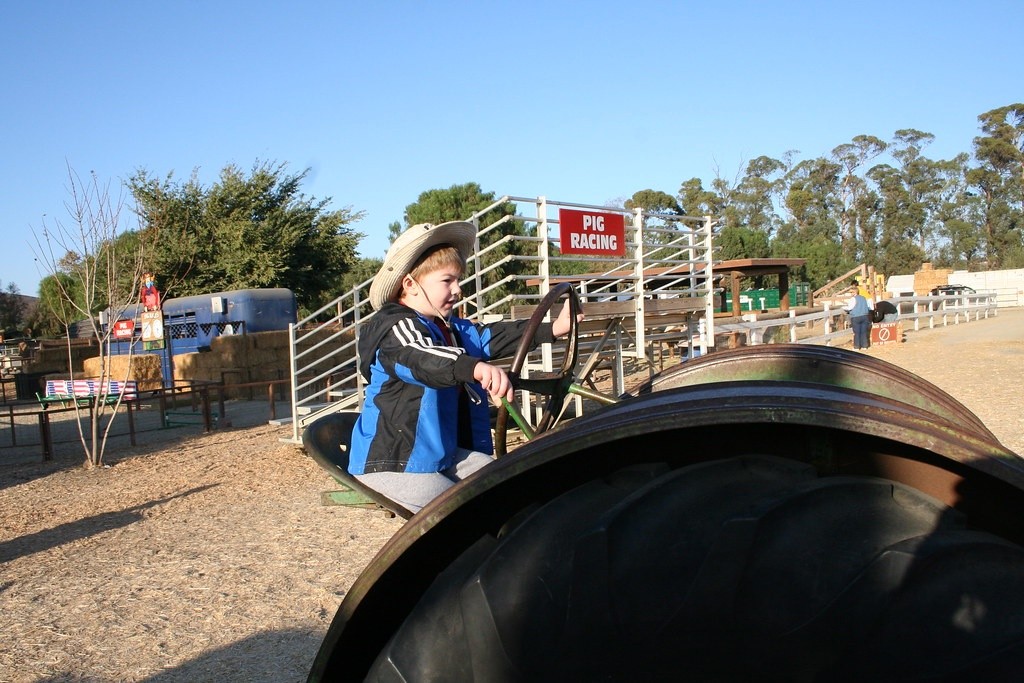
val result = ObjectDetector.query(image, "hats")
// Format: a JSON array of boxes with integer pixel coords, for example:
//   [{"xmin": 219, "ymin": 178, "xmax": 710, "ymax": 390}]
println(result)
[{"xmin": 369, "ymin": 220, "xmax": 476, "ymax": 313}]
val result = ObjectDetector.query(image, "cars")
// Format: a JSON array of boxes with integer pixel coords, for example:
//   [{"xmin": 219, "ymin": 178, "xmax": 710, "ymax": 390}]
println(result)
[{"xmin": 926, "ymin": 284, "xmax": 976, "ymax": 310}]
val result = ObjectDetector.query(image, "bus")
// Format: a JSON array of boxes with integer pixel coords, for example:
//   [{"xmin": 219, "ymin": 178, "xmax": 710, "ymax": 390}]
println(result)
[{"xmin": 99, "ymin": 287, "xmax": 297, "ymax": 388}]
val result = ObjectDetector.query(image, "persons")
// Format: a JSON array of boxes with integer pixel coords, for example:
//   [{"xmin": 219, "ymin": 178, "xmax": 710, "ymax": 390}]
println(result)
[
  {"xmin": 20, "ymin": 327, "xmax": 32, "ymax": 358},
  {"xmin": 347, "ymin": 221, "xmax": 586, "ymax": 515},
  {"xmin": 841, "ymin": 280, "xmax": 898, "ymax": 351}
]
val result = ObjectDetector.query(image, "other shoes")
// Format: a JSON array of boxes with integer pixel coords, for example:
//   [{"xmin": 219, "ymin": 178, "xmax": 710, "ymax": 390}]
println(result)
[
  {"xmin": 861, "ymin": 348, "xmax": 867, "ymax": 350},
  {"xmin": 852, "ymin": 349, "xmax": 859, "ymax": 351}
]
